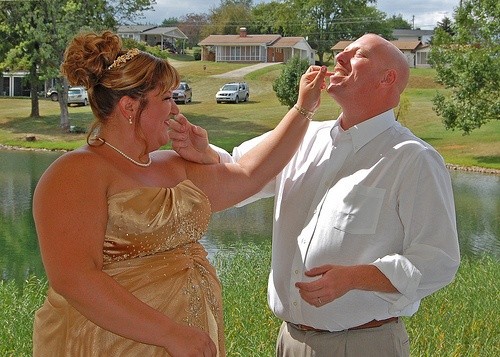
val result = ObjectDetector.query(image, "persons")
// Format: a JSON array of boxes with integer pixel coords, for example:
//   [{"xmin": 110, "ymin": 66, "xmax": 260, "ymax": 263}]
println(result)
[
  {"xmin": 168, "ymin": 33, "xmax": 460, "ymax": 357},
  {"xmin": 32, "ymin": 31, "xmax": 336, "ymax": 357}
]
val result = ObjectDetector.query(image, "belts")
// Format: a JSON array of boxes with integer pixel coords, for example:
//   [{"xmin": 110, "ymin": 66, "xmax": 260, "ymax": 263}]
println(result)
[{"xmin": 289, "ymin": 316, "xmax": 397, "ymax": 332}]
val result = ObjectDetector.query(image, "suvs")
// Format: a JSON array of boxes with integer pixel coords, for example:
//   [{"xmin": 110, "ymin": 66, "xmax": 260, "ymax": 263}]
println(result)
[{"xmin": 216, "ymin": 81, "xmax": 250, "ymax": 104}]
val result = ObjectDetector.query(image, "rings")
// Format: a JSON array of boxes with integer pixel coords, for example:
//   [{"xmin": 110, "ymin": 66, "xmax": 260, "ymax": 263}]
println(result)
[{"xmin": 317, "ymin": 297, "xmax": 322, "ymax": 304}]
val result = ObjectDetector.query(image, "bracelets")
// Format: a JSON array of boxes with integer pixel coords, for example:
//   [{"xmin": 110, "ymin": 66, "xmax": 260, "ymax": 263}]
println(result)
[{"xmin": 294, "ymin": 103, "xmax": 315, "ymax": 121}]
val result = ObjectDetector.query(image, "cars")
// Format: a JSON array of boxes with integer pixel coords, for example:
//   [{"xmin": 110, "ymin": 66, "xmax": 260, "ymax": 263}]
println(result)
[
  {"xmin": 171, "ymin": 83, "xmax": 193, "ymax": 105},
  {"xmin": 68, "ymin": 87, "xmax": 89, "ymax": 107}
]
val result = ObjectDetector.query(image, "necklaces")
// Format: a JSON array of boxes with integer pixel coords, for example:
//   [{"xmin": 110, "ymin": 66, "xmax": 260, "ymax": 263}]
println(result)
[{"xmin": 95, "ymin": 135, "xmax": 152, "ymax": 167}]
